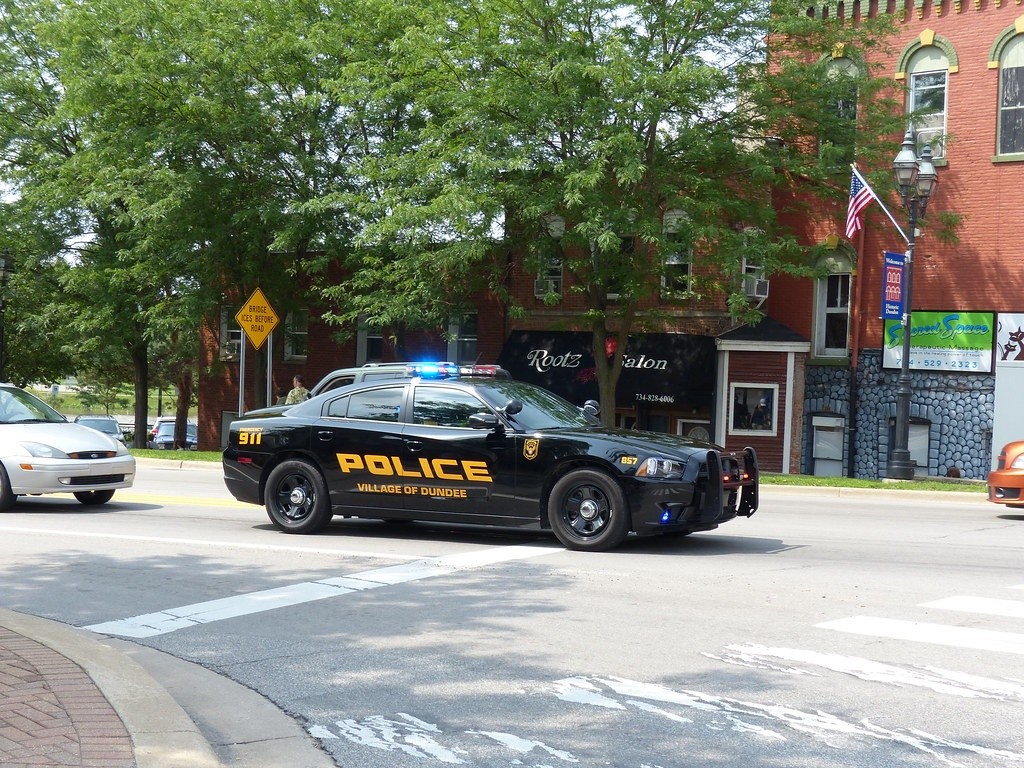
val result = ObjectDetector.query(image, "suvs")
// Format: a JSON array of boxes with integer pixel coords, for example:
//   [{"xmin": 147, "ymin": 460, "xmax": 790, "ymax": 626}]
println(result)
[{"xmin": 309, "ymin": 361, "xmax": 456, "ymax": 382}]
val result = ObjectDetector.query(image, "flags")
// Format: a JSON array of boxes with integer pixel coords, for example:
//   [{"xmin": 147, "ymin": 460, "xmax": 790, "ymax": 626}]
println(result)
[{"xmin": 846, "ymin": 172, "xmax": 875, "ymax": 239}]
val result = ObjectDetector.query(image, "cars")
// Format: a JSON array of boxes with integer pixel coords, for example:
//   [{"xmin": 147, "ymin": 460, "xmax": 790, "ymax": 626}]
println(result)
[
  {"xmin": 75, "ymin": 415, "xmax": 127, "ymax": 446},
  {"xmin": 0, "ymin": 381, "xmax": 136, "ymax": 507},
  {"xmin": 151, "ymin": 423, "xmax": 198, "ymax": 451},
  {"xmin": 987, "ymin": 441, "xmax": 1024, "ymax": 506},
  {"xmin": 148, "ymin": 418, "xmax": 190, "ymax": 440},
  {"xmin": 222, "ymin": 360, "xmax": 761, "ymax": 551}
]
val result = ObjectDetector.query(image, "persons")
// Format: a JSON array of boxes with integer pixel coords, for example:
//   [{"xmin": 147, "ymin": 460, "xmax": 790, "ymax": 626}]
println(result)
[
  {"xmin": 734, "ymin": 396, "xmax": 748, "ymax": 429},
  {"xmin": 751, "ymin": 398, "xmax": 770, "ymax": 430},
  {"xmin": 273, "ymin": 387, "xmax": 286, "ymax": 405},
  {"xmin": 285, "ymin": 374, "xmax": 310, "ymax": 405}
]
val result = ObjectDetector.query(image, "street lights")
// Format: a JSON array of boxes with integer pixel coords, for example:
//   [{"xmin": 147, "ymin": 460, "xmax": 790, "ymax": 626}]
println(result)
[{"xmin": 893, "ymin": 128, "xmax": 937, "ymax": 477}]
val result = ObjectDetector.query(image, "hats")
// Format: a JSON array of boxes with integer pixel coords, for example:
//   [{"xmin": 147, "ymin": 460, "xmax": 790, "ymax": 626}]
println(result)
[{"xmin": 760, "ymin": 399, "xmax": 766, "ymax": 407}]
